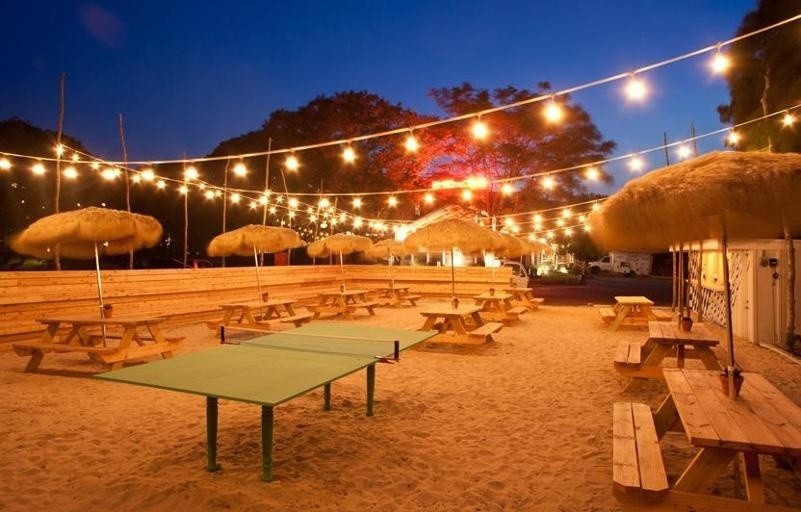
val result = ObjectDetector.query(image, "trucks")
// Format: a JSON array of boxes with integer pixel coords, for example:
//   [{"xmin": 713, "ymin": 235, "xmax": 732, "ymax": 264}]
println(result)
[
  {"xmin": 500, "ymin": 258, "xmax": 530, "ymax": 289},
  {"xmin": 587, "ymin": 249, "xmax": 654, "ymax": 279}
]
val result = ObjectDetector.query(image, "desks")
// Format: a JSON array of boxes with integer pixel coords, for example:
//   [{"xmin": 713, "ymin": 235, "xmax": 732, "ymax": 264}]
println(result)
[
  {"xmin": 419, "ymin": 286, "xmax": 533, "ymax": 351},
  {"xmin": 610, "ymin": 295, "xmax": 658, "ymax": 332},
  {"xmin": 642, "ymin": 320, "xmax": 801, "ymax": 511},
  {"xmin": 24, "ymin": 313, "xmax": 174, "ymax": 373},
  {"xmin": 219, "ymin": 286, "xmax": 417, "ymax": 339},
  {"xmin": 95, "ymin": 318, "xmax": 440, "ymax": 484}
]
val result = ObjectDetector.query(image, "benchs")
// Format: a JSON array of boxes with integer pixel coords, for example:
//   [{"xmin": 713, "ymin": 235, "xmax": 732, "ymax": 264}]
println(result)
[
  {"xmin": 402, "ymin": 293, "xmax": 422, "ymax": 306},
  {"xmin": 207, "ymin": 313, "xmax": 265, "ymax": 338},
  {"xmin": 12, "ymin": 332, "xmax": 187, "ymax": 374},
  {"xmin": 530, "ymin": 297, "xmax": 545, "ymax": 311},
  {"xmin": 598, "ymin": 307, "xmax": 674, "ymax": 334},
  {"xmin": 611, "ymin": 340, "xmax": 670, "ymax": 511}
]
